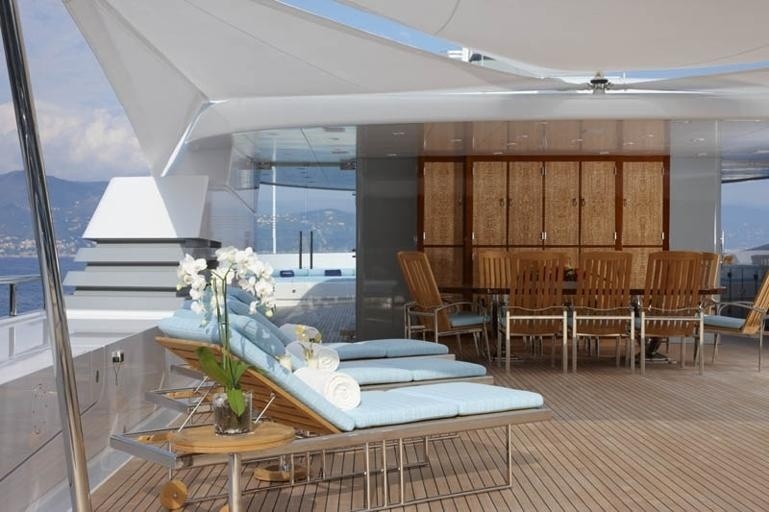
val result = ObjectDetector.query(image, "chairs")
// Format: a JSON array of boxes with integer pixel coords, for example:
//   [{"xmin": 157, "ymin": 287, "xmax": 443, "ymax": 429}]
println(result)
[
  {"xmin": 177, "ymin": 295, "xmax": 495, "ymax": 467},
  {"xmin": 111, "ymin": 316, "xmax": 554, "ymax": 510},
  {"xmin": 470, "ymin": 251, "xmax": 719, "ymax": 375},
  {"xmin": 692, "ymin": 272, "xmax": 768, "ymax": 372},
  {"xmin": 400, "ymin": 252, "xmax": 491, "ymax": 363},
  {"xmin": 200, "ymin": 288, "xmax": 458, "ymax": 361}
]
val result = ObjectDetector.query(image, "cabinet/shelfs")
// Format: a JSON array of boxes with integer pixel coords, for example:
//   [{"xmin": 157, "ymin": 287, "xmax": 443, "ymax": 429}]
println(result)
[
  {"xmin": 473, "ymin": 161, "xmax": 620, "ymax": 247},
  {"xmin": 622, "ymin": 162, "xmax": 663, "ymax": 246},
  {"xmin": 424, "ymin": 162, "xmax": 464, "ymax": 246}
]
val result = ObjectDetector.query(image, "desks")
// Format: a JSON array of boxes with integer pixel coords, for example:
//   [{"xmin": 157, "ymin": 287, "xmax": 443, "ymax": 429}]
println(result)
[{"xmin": 434, "ymin": 282, "xmax": 727, "ymax": 367}]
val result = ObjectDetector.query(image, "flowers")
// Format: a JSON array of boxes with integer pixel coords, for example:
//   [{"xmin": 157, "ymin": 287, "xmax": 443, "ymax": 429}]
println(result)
[{"xmin": 176, "ymin": 244, "xmax": 280, "ymax": 416}]
